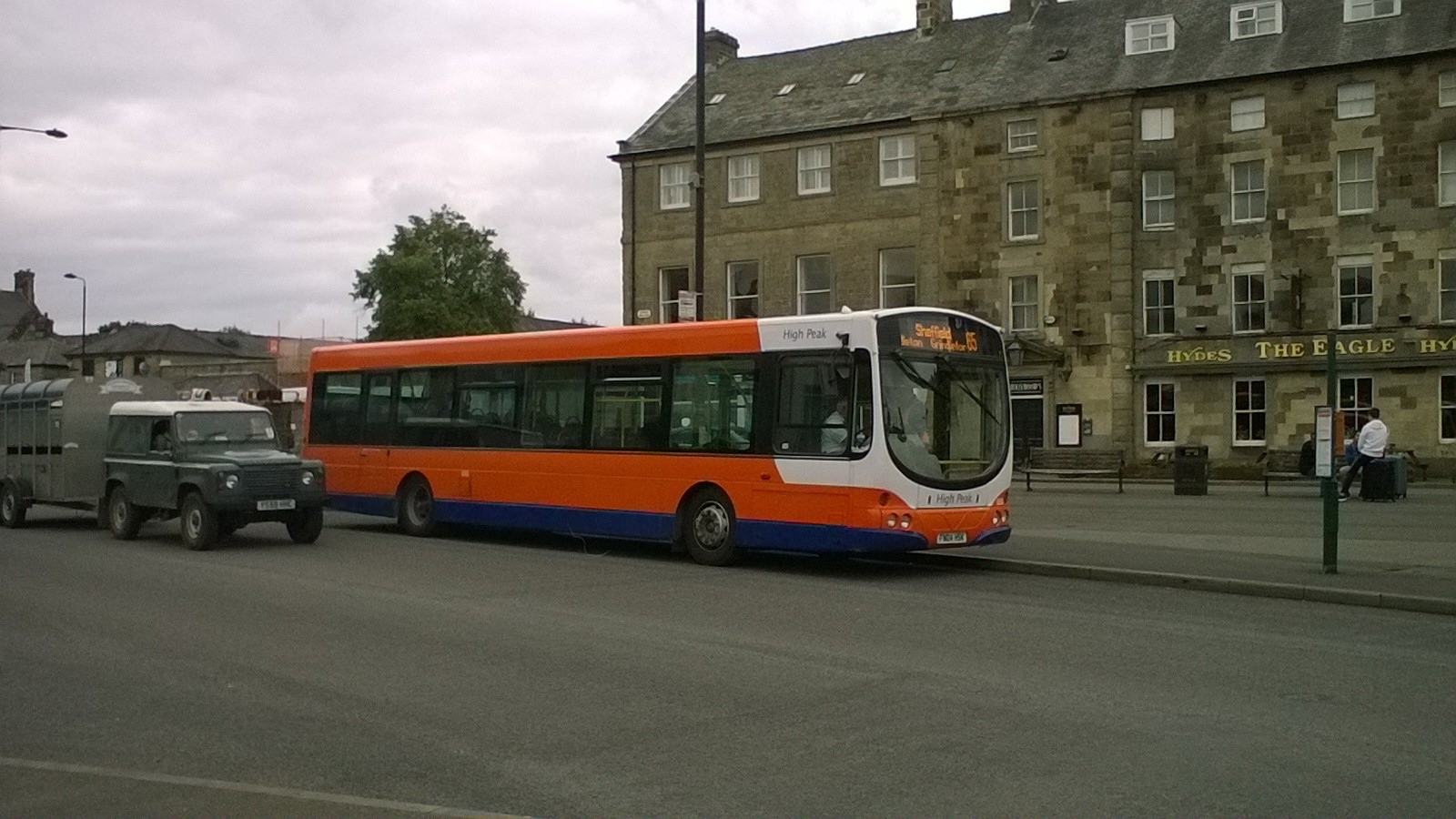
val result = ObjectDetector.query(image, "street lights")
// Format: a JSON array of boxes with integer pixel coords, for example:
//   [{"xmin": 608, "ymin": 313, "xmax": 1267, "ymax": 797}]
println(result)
[{"xmin": 63, "ymin": 272, "xmax": 88, "ymax": 375}]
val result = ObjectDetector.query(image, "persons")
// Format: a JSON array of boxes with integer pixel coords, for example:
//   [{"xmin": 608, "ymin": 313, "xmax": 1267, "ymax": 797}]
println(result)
[
  {"xmin": 1337, "ymin": 407, "xmax": 1388, "ymax": 503},
  {"xmin": 746, "ymin": 279, "xmax": 758, "ymax": 295},
  {"xmin": 898, "ymin": 384, "xmax": 931, "ymax": 453},
  {"xmin": 819, "ymin": 398, "xmax": 850, "ymax": 456},
  {"xmin": 154, "ymin": 418, "xmax": 181, "ymax": 451},
  {"xmin": 1299, "ymin": 432, "xmax": 1316, "ymax": 475}
]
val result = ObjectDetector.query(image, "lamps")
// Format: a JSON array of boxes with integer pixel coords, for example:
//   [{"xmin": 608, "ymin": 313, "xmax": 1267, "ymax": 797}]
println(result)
[
  {"xmin": 1400, "ymin": 314, "xmax": 1411, "ymax": 324},
  {"xmin": 1008, "ymin": 342, "xmax": 1025, "ymax": 366},
  {"xmin": 1071, "ymin": 328, "xmax": 1085, "ymax": 337},
  {"xmin": 1194, "ymin": 324, "xmax": 1207, "ymax": 334}
]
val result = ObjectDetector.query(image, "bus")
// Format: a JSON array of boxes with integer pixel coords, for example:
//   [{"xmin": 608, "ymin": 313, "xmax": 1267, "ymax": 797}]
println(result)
[{"xmin": 300, "ymin": 307, "xmax": 1016, "ymax": 567}]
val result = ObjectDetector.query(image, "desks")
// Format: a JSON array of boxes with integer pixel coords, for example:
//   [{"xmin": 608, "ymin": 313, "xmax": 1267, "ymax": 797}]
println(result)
[{"xmin": 1383, "ymin": 448, "xmax": 1430, "ymax": 482}]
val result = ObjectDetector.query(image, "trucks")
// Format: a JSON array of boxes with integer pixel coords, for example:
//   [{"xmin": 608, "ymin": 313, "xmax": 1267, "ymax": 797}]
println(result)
[{"xmin": 0, "ymin": 376, "xmax": 326, "ymax": 552}]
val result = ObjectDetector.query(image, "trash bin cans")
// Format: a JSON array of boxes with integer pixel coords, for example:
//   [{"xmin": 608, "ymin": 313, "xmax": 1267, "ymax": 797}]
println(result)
[
  {"xmin": 1175, "ymin": 445, "xmax": 1209, "ymax": 496},
  {"xmin": 1386, "ymin": 455, "xmax": 1408, "ymax": 500}
]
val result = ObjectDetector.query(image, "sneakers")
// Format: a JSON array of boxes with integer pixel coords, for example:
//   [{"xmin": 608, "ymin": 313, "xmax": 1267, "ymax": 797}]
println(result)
[{"xmin": 1338, "ymin": 493, "xmax": 1350, "ymax": 502}]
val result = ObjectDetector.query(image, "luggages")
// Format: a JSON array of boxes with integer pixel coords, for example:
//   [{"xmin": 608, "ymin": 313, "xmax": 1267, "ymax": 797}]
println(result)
[{"xmin": 1363, "ymin": 455, "xmax": 1407, "ymax": 502}]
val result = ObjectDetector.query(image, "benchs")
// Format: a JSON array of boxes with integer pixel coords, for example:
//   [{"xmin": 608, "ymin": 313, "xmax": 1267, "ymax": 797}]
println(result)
[
  {"xmin": 1262, "ymin": 449, "xmax": 1349, "ymax": 496},
  {"xmin": 1406, "ymin": 461, "xmax": 1428, "ymax": 475},
  {"xmin": 1022, "ymin": 447, "xmax": 1124, "ymax": 494}
]
